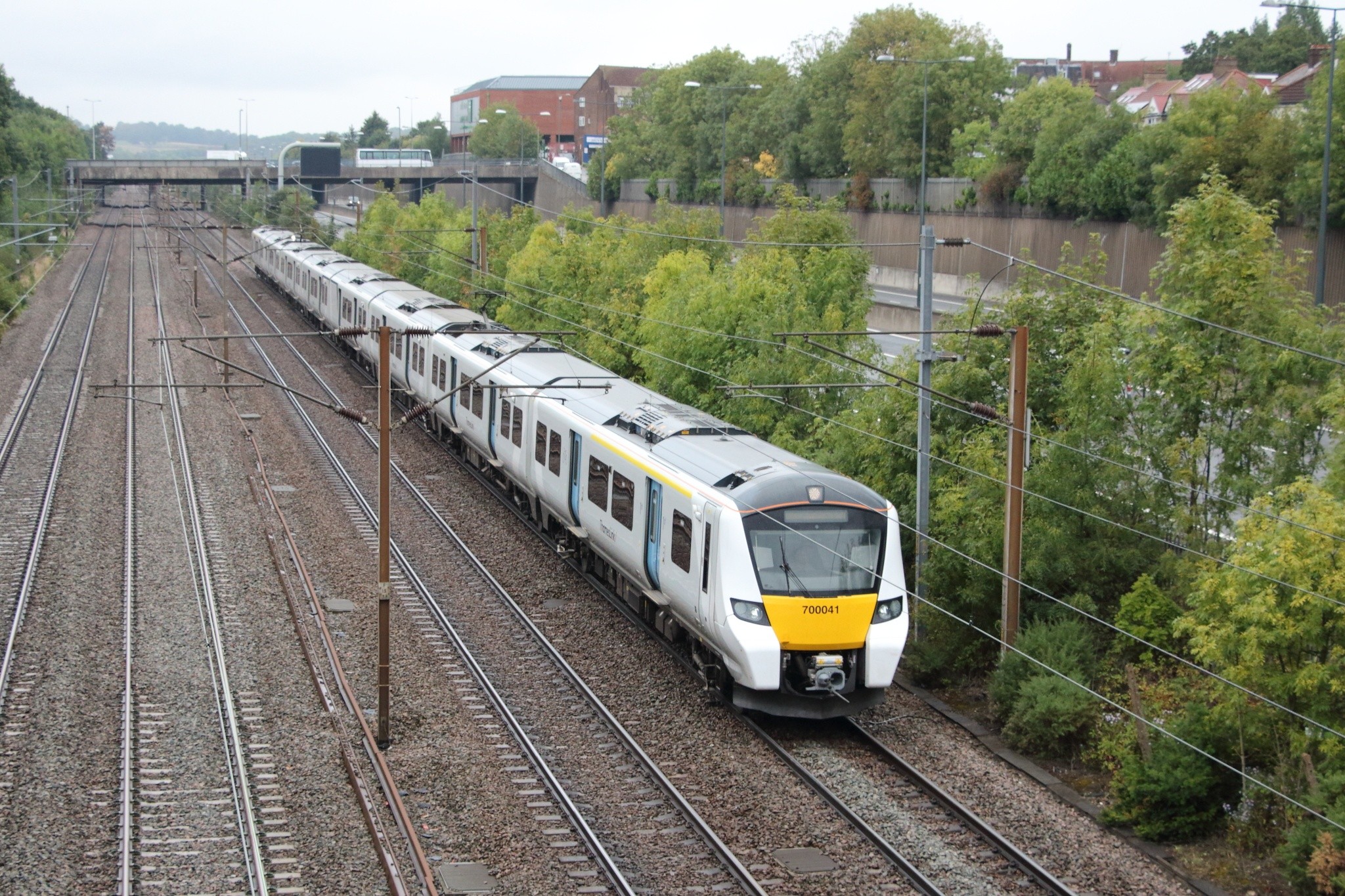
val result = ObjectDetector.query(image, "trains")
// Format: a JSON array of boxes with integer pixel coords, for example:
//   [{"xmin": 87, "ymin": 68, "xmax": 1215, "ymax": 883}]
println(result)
[{"xmin": 250, "ymin": 225, "xmax": 910, "ymax": 721}]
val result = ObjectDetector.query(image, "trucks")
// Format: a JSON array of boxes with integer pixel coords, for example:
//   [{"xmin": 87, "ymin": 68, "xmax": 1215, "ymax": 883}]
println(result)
[{"xmin": 206, "ymin": 150, "xmax": 247, "ymax": 161}]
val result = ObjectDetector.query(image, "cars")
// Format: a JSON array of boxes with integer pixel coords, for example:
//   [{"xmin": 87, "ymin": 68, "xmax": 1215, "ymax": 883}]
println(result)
[{"xmin": 347, "ymin": 196, "xmax": 360, "ymax": 206}]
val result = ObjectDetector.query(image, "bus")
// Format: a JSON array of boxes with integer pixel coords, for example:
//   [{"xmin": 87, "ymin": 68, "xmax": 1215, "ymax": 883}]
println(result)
[{"xmin": 354, "ymin": 149, "xmax": 434, "ymax": 168}]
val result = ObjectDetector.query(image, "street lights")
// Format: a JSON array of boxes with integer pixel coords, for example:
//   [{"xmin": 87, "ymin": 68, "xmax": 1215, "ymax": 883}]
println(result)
[
  {"xmin": 874, "ymin": 54, "xmax": 976, "ymax": 307},
  {"xmin": 405, "ymin": 95, "xmax": 418, "ymax": 148},
  {"xmin": 495, "ymin": 109, "xmax": 552, "ymax": 204},
  {"xmin": 1259, "ymin": 0, "xmax": 1339, "ymax": 305},
  {"xmin": 239, "ymin": 98, "xmax": 257, "ymax": 161},
  {"xmin": 685, "ymin": 81, "xmax": 762, "ymax": 237},
  {"xmin": 396, "ymin": 107, "xmax": 401, "ymax": 166},
  {"xmin": 84, "ymin": 98, "xmax": 104, "ymax": 159},
  {"xmin": 573, "ymin": 98, "xmax": 632, "ymax": 217},
  {"xmin": 434, "ymin": 120, "xmax": 488, "ymax": 206}
]
